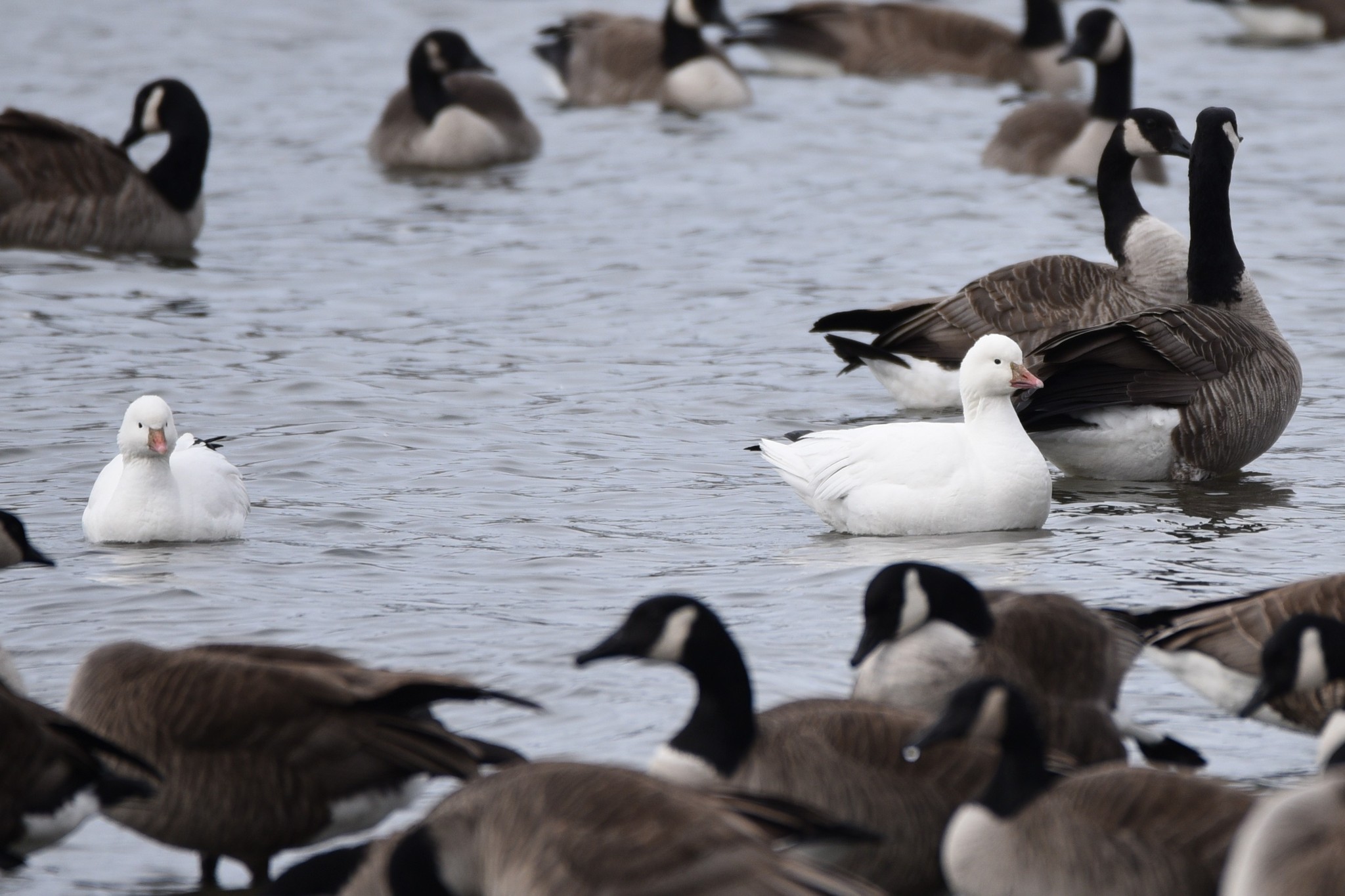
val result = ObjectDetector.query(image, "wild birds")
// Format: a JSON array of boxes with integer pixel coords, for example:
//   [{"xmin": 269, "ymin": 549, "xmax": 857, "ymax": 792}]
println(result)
[
  {"xmin": 0, "ymin": 506, "xmax": 1344, "ymax": 896},
  {"xmin": 538, "ymin": 0, "xmax": 1344, "ymax": 186},
  {"xmin": 0, "ymin": 79, "xmax": 211, "ymax": 255},
  {"xmin": 82, "ymin": 395, "xmax": 248, "ymax": 544},
  {"xmin": 371, "ymin": 27, "xmax": 546, "ymax": 170},
  {"xmin": 760, "ymin": 335, "xmax": 1054, "ymax": 536},
  {"xmin": 810, "ymin": 107, "xmax": 1302, "ymax": 484}
]
